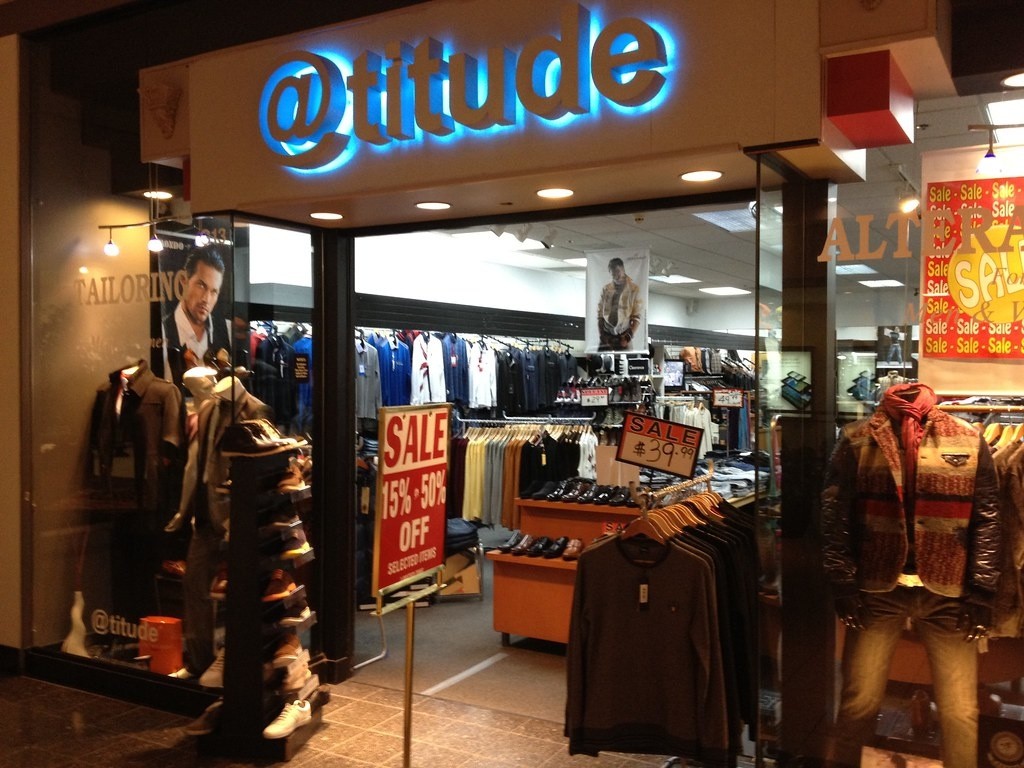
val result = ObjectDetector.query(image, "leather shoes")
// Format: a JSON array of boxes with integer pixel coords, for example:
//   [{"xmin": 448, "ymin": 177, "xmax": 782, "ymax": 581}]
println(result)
[
  {"xmin": 533, "ymin": 481, "xmax": 644, "ymax": 507},
  {"xmin": 498, "ymin": 530, "xmax": 581, "ymax": 560}
]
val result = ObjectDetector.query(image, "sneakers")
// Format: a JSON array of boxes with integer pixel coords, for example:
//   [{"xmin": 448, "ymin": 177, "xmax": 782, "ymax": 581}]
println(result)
[
  {"xmin": 279, "ymin": 529, "xmax": 308, "ymax": 558},
  {"xmin": 278, "ymin": 604, "xmax": 311, "ymax": 627},
  {"xmin": 200, "ymin": 646, "xmax": 223, "ymax": 685},
  {"xmin": 260, "ymin": 568, "xmax": 296, "ymax": 601},
  {"xmin": 264, "ymin": 701, "xmax": 312, "ymax": 739},
  {"xmin": 276, "ymin": 635, "xmax": 304, "ymax": 656},
  {"xmin": 283, "ymin": 667, "xmax": 312, "ymax": 691},
  {"xmin": 275, "ymin": 455, "xmax": 313, "ymax": 492}
]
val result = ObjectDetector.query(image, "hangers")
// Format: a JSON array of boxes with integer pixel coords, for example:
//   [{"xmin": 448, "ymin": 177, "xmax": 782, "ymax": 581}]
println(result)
[
  {"xmin": 622, "ymin": 474, "xmax": 727, "ymax": 566},
  {"xmin": 462, "ymin": 418, "xmax": 598, "ymax": 445},
  {"xmin": 656, "ymin": 395, "xmax": 707, "ymax": 413},
  {"xmin": 251, "ymin": 321, "xmax": 578, "ymax": 357},
  {"xmin": 972, "ymin": 405, "xmax": 1024, "ymax": 449}
]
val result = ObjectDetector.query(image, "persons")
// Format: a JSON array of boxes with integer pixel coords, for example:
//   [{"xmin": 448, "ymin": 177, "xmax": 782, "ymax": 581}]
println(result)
[
  {"xmin": 597, "ymin": 258, "xmax": 643, "ymax": 352},
  {"xmin": 884, "ymin": 327, "xmax": 907, "ymax": 365},
  {"xmin": 820, "ymin": 384, "xmax": 1003, "ymax": 768},
  {"xmin": 88, "ymin": 365, "xmax": 271, "ymax": 679},
  {"xmin": 162, "ymin": 248, "xmax": 230, "ymax": 399}
]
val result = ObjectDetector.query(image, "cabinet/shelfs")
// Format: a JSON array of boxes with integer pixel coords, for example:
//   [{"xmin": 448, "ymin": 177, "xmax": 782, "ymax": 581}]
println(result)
[
  {"xmin": 485, "ymin": 499, "xmax": 641, "ymax": 647},
  {"xmin": 217, "ymin": 419, "xmax": 331, "ymax": 764}
]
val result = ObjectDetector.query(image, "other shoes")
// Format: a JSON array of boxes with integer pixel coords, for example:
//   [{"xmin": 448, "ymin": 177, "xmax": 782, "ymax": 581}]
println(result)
[{"xmin": 220, "ymin": 417, "xmax": 306, "ymax": 457}]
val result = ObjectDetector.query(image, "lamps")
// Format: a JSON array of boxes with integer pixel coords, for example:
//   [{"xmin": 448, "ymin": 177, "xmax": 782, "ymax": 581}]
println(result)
[{"xmin": 98, "ymin": 161, "xmax": 213, "ymax": 257}]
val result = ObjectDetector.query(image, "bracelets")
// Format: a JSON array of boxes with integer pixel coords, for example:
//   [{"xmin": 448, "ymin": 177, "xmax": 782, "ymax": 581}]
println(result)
[{"xmin": 625, "ymin": 332, "xmax": 633, "ymax": 342}]
